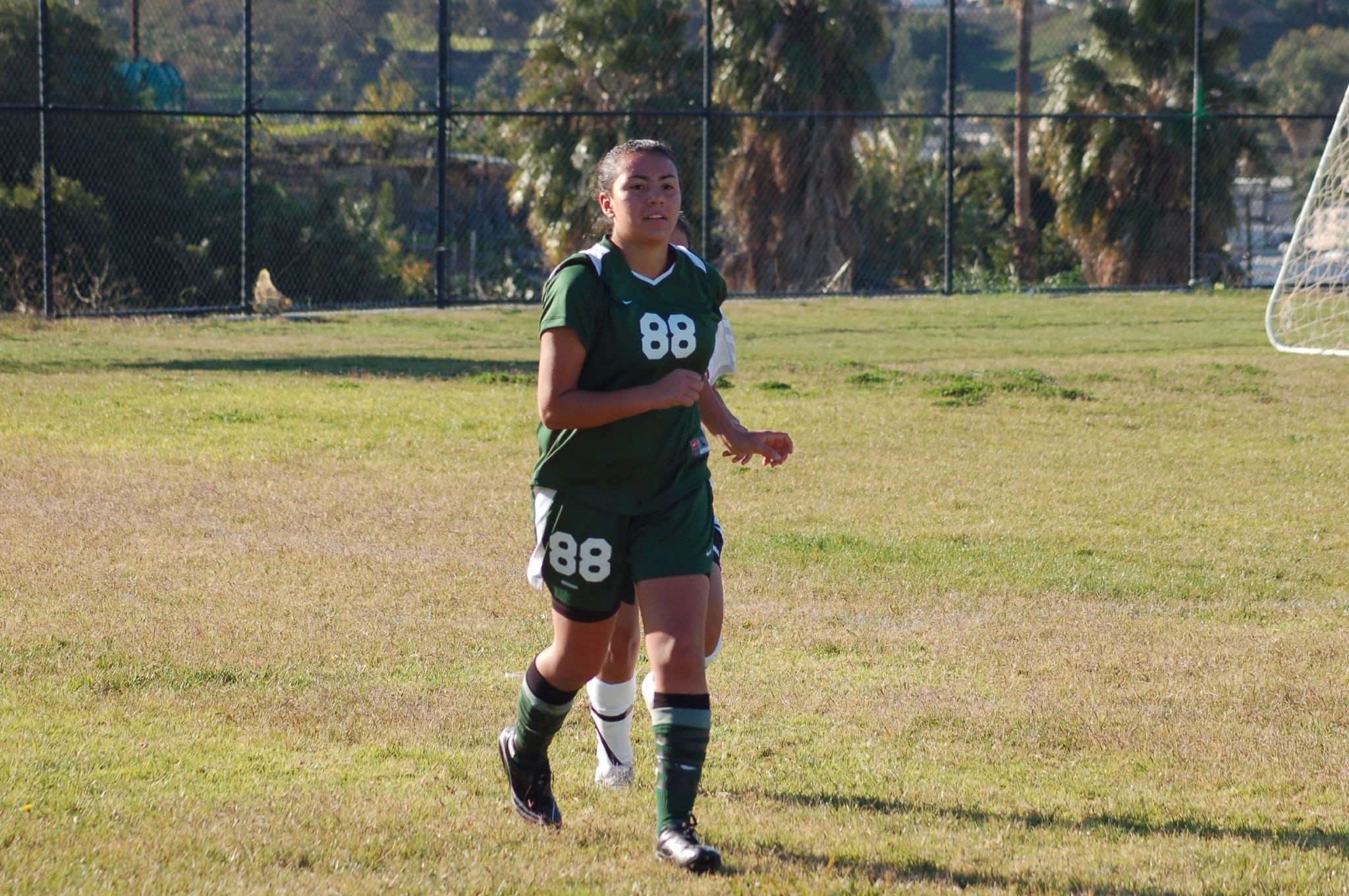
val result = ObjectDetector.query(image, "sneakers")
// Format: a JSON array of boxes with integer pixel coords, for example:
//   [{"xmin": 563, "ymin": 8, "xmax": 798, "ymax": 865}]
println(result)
[
  {"xmin": 655, "ymin": 813, "xmax": 722, "ymax": 875},
  {"xmin": 498, "ymin": 727, "xmax": 563, "ymax": 830},
  {"xmin": 641, "ymin": 673, "xmax": 656, "ymax": 714},
  {"xmin": 596, "ymin": 761, "xmax": 632, "ymax": 787}
]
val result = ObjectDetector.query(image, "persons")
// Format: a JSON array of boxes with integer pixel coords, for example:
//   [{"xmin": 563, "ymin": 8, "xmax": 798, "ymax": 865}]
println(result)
[{"xmin": 500, "ymin": 136, "xmax": 795, "ymax": 871}]
